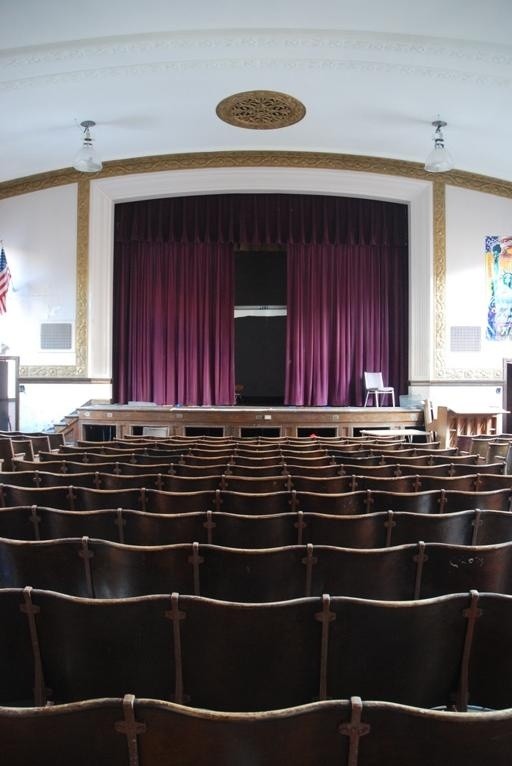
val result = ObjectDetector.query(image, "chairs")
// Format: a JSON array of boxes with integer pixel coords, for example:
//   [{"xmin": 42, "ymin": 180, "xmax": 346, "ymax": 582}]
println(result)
[{"xmin": 363, "ymin": 371, "xmax": 395, "ymax": 407}]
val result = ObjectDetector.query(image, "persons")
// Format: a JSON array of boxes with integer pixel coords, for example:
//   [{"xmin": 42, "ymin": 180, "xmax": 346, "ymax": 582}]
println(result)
[{"xmin": 486, "ymin": 244, "xmax": 512, "ymax": 341}]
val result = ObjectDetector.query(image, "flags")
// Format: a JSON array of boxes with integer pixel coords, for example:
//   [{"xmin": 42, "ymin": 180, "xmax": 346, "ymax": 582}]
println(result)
[{"xmin": 0, "ymin": 248, "xmax": 11, "ymax": 315}]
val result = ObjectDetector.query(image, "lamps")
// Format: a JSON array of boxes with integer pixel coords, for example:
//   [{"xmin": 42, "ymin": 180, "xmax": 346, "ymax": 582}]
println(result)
[
  {"xmin": 424, "ymin": 121, "xmax": 454, "ymax": 173},
  {"xmin": 73, "ymin": 119, "xmax": 103, "ymax": 172}
]
